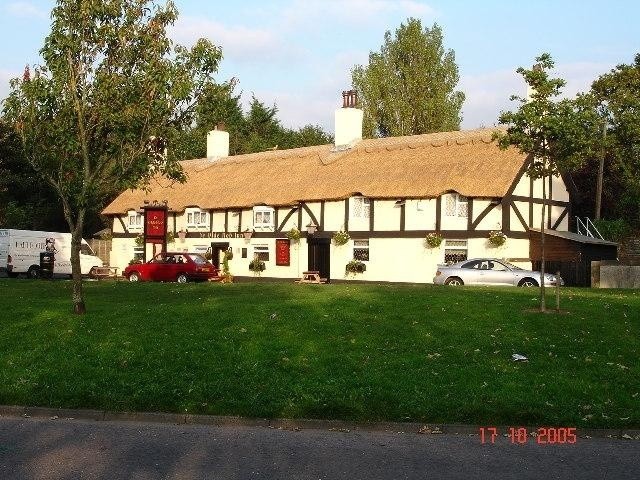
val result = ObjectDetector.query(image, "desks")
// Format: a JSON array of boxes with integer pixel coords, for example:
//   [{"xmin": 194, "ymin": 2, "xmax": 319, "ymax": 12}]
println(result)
[{"xmin": 93, "ymin": 266, "xmax": 120, "ymax": 280}]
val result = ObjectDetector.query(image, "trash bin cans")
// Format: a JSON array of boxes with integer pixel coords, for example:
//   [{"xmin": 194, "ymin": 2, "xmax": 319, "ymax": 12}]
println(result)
[{"xmin": 40, "ymin": 252, "xmax": 54, "ymax": 279}]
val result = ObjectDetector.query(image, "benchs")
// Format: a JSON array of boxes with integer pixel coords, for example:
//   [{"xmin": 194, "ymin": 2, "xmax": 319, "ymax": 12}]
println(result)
[
  {"xmin": 91, "ymin": 271, "xmax": 120, "ymax": 281},
  {"xmin": 295, "ymin": 277, "xmax": 327, "ymax": 284}
]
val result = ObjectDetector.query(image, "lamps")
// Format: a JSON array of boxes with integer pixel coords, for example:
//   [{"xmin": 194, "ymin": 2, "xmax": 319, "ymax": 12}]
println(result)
[
  {"xmin": 244, "ymin": 228, "xmax": 253, "ymax": 241},
  {"xmin": 395, "ymin": 200, "xmax": 405, "ymax": 205},
  {"xmin": 178, "ymin": 230, "xmax": 188, "ymax": 241},
  {"xmin": 306, "ymin": 219, "xmax": 319, "ymax": 241}
]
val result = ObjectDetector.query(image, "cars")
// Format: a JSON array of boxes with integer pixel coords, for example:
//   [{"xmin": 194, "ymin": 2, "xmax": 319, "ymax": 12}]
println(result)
[
  {"xmin": 125, "ymin": 252, "xmax": 218, "ymax": 283},
  {"xmin": 433, "ymin": 258, "xmax": 564, "ymax": 288}
]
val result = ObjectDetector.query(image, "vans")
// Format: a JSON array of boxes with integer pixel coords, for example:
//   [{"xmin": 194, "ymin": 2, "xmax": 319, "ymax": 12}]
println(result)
[{"xmin": 0, "ymin": 229, "xmax": 103, "ymax": 278}]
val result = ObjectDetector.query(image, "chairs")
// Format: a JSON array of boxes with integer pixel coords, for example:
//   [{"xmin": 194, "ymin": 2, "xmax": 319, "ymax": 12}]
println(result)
[
  {"xmin": 177, "ymin": 256, "xmax": 183, "ymax": 264},
  {"xmin": 165, "ymin": 257, "xmax": 176, "ymax": 264}
]
